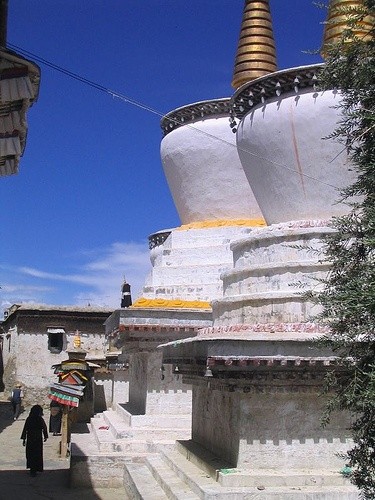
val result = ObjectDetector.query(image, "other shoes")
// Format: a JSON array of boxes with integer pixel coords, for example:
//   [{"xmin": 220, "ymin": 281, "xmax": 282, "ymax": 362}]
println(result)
[{"xmin": 30, "ymin": 472, "xmax": 37, "ymax": 477}]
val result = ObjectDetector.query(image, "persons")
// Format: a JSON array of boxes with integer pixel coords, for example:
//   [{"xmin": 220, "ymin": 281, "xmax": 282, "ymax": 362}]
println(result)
[
  {"xmin": 10, "ymin": 383, "xmax": 27, "ymax": 421},
  {"xmin": 20, "ymin": 404, "xmax": 49, "ymax": 477}
]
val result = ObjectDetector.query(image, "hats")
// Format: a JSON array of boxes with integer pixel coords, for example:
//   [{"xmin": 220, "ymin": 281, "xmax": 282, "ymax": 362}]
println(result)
[{"xmin": 16, "ymin": 384, "xmax": 21, "ymax": 386}]
